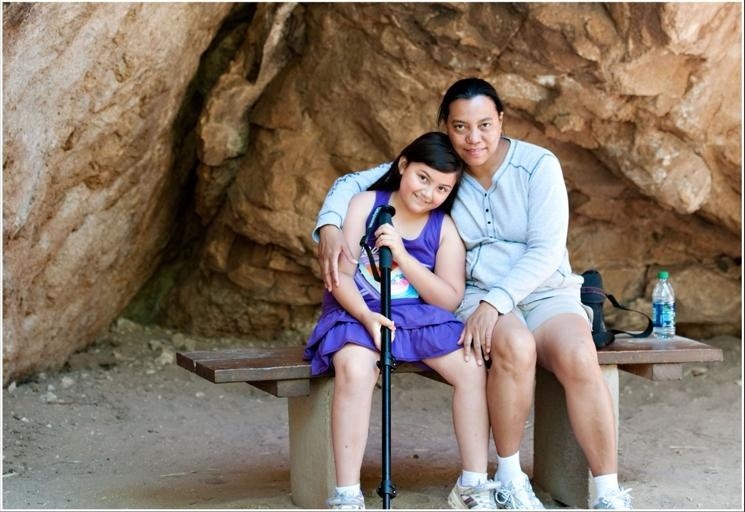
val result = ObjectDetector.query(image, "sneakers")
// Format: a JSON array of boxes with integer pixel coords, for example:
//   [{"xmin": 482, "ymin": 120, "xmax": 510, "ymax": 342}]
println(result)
[
  {"xmin": 494, "ymin": 469, "xmax": 544, "ymax": 509},
  {"xmin": 447, "ymin": 474, "xmax": 499, "ymax": 508},
  {"xmin": 326, "ymin": 489, "xmax": 365, "ymax": 509},
  {"xmin": 586, "ymin": 485, "xmax": 634, "ymax": 508}
]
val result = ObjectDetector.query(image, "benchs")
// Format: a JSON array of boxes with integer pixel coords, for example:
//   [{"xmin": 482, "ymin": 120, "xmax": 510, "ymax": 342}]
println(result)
[{"xmin": 175, "ymin": 326, "xmax": 724, "ymax": 509}]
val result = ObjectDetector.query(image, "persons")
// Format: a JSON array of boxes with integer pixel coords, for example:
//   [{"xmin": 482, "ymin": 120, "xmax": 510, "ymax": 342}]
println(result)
[
  {"xmin": 303, "ymin": 132, "xmax": 502, "ymax": 510},
  {"xmin": 312, "ymin": 79, "xmax": 632, "ymax": 509}
]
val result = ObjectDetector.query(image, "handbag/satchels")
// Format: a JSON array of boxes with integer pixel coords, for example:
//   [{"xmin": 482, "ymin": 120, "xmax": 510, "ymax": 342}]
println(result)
[{"xmin": 579, "ymin": 270, "xmax": 612, "ymax": 350}]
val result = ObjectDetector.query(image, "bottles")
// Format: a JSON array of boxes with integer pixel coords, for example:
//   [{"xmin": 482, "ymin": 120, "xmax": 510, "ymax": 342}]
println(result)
[{"xmin": 650, "ymin": 271, "xmax": 677, "ymax": 342}]
